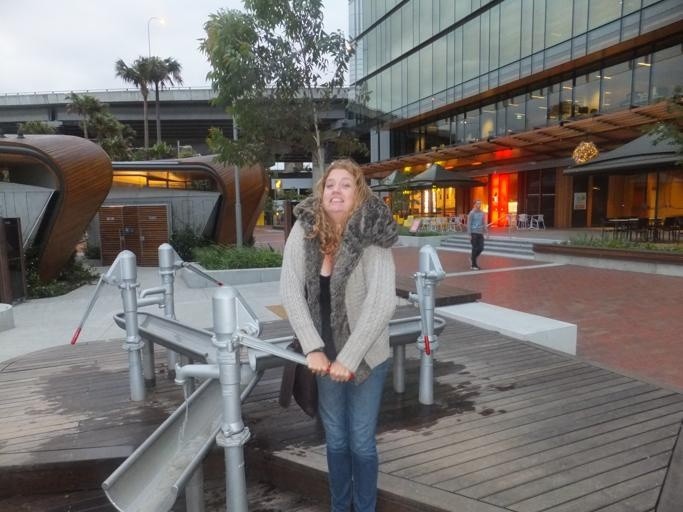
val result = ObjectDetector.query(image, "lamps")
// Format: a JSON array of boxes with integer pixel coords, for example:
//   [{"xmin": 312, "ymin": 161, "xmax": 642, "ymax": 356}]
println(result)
[{"xmin": 572, "ymin": 134, "xmax": 599, "ymax": 165}]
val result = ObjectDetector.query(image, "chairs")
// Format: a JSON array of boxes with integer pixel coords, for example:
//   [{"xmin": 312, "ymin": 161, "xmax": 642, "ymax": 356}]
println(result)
[
  {"xmin": 409, "ymin": 213, "xmax": 545, "ymax": 237},
  {"xmin": 605, "ymin": 215, "xmax": 683, "ymax": 243}
]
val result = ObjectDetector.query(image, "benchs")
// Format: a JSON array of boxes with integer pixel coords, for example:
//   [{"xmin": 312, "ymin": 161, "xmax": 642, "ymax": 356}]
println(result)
[{"xmin": 619, "ymin": 90, "xmax": 664, "ymax": 108}]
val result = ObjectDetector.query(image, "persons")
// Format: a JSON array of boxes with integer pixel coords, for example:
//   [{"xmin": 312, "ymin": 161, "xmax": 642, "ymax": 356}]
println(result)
[
  {"xmin": 280, "ymin": 160, "xmax": 397, "ymax": 511},
  {"xmin": 467, "ymin": 201, "xmax": 488, "ymax": 271}
]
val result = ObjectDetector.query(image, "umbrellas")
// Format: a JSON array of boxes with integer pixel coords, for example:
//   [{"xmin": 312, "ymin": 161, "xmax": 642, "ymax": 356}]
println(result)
[
  {"xmin": 403, "ymin": 164, "xmax": 484, "ymax": 216},
  {"xmin": 368, "ymin": 169, "xmax": 430, "ymax": 191},
  {"xmin": 562, "ymin": 122, "xmax": 682, "ymax": 237}
]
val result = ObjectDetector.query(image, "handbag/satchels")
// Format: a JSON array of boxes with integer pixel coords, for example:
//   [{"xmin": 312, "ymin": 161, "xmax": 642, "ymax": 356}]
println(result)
[{"xmin": 279, "ymin": 338, "xmax": 318, "ymax": 418}]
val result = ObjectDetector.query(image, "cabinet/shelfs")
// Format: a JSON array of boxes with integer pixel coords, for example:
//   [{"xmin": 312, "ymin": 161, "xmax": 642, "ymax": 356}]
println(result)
[{"xmin": 98, "ymin": 203, "xmax": 172, "ymax": 267}]
